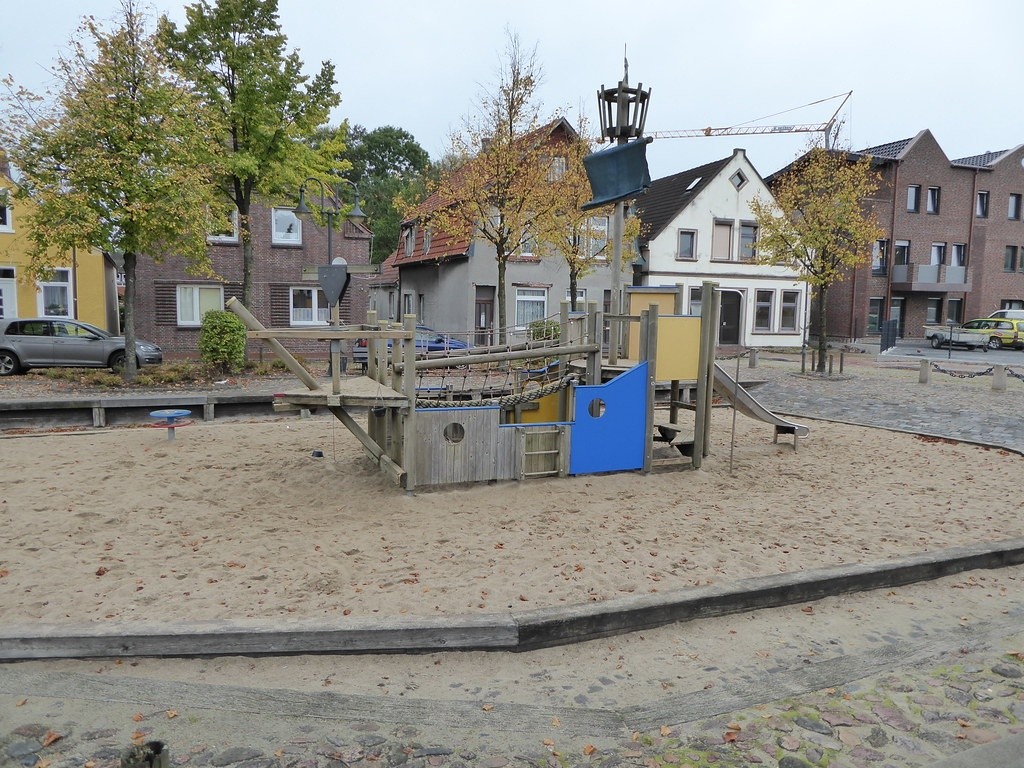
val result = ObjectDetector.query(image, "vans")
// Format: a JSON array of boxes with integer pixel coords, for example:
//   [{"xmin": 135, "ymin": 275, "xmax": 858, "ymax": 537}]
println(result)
[
  {"xmin": 927, "ymin": 308, "xmax": 955, "ymax": 324},
  {"xmin": 971, "ymin": 310, "xmax": 1024, "ymax": 330}
]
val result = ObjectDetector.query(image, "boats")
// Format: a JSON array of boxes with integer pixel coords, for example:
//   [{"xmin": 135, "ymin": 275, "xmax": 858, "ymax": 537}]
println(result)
[{"xmin": 923, "ymin": 325, "xmax": 998, "ymax": 343}]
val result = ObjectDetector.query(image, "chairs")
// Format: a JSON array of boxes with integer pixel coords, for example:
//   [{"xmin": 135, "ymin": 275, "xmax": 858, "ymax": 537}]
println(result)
[{"xmin": 58, "ymin": 324, "xmax": 68, "ymax": 334}]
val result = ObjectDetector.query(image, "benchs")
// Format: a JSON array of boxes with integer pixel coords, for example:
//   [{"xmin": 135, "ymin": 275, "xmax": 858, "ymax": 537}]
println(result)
[{"xmin": 352, "ymin": 347, "xmax": 427, "ymax": 377}]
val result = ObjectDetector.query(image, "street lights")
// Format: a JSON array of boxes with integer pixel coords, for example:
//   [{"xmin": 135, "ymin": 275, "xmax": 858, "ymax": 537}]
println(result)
[
  {"xmin": 597, "ymin": 41, "xmax": 654, "ymax": 363},
  {"xmin": 292, "ymin": 177, "xmax": 370, "ymax": 375}
]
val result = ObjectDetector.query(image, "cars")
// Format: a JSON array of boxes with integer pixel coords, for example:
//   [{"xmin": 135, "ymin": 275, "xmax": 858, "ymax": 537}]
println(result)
[
  {"xmin": 959, "ymin": 319, "xmax": 1024, "ymax": 349},
  {"xmin": 353, "ymin": 320, "xmax": 477, "ymax": 373},
  {"xmin": 0, "ymin": 315, "xmax": 161, "ymax": 379}
]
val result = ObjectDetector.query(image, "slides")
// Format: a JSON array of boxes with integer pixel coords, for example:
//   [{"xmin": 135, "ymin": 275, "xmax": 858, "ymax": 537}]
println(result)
[{"xmin": 714, "ymin": 362, "xmax": 809, "ymax": 437}]
